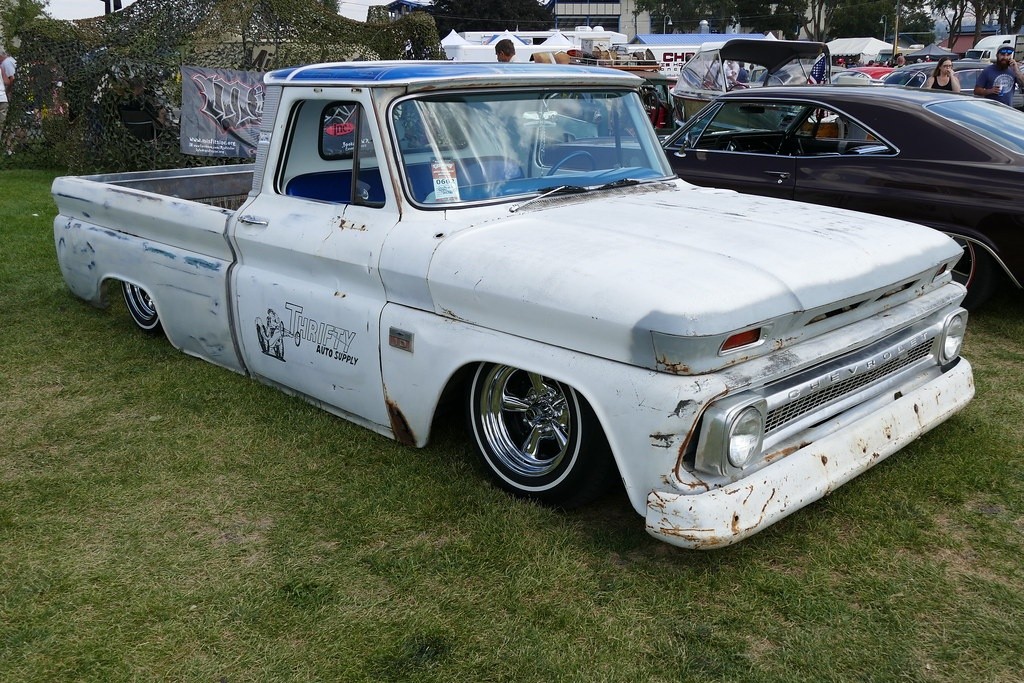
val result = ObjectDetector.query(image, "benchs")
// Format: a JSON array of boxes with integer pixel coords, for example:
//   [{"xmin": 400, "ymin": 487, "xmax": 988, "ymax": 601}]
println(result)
[{"xmin": 285, "ymin": 155, "xmax": 525, "ymax": 203}]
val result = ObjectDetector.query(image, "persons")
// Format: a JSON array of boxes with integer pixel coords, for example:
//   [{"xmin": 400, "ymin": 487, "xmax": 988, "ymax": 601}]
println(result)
[
  {"xmin": 923, "ymin": 55, "xmax": 961, "ymax": 93},
  {"xmin": 495, "ymin": 39, "xmax": 534, "ymax": 119},
  {"xmin": 0, "ymin": 47, "xmax": 66, "ymax": 157},
  {"xmin": 917, "ymin": 55, "xmax": 934, "ymax": 62},
  {"xmin": 837, "ymin": 57, "xmax": 884, "ymax": 69},
  {"xmin": 894, "ymin": 56, "xmax": 906, "ymax": 68},
  {"xmin": 973, "ymin": 43, "xmax": 1024, "ymax": 108},
  {"xmin": 711, "ymin": 58, "xmax": 757, "ymax": 87}
]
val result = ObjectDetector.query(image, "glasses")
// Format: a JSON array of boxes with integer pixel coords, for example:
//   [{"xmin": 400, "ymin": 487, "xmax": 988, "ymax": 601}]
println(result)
[
  {"xmin": 998, "ymin": 49, "xmax": 1014, "ymax": 55},
  {"xmin": 942, "ymin": 65, "xmax": 952, "ymax": 68}
]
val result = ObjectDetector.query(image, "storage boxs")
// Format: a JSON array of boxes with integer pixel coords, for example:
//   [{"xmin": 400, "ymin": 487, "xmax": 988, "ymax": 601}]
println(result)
[
  {"xmin": 593, "ymin": 51, "xmax": 637, "ymax": 65},
  {"xmin": 567, "ymin": 50, "xmax": 584, "ymax": 63}
]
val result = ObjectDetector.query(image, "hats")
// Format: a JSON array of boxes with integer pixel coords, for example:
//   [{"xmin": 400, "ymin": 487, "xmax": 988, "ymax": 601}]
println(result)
[
  {"xmin": 926, "ymin": 56, "xmax": 929, "ymax": 58},
  {"xmin": 996, "ymin": 44, "xmax": 1015, "ymax": 55}
]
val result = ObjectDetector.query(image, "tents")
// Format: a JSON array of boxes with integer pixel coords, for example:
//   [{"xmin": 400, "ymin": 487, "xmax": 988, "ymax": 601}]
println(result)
[{"xmin": 826, "ymin": 37, "xmax": 894, "ymax": 64}]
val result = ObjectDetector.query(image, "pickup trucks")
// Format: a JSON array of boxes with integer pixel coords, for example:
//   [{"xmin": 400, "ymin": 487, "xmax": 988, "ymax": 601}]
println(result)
[{"xmin": 47, "ymin": 60, "xmax": 980, "ymax": 551}]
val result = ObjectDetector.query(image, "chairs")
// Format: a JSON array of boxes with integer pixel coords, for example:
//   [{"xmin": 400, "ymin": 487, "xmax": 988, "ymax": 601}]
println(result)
[{"xmin": 119, "ymin": 102, "xmax": 180, "ymax": 172}]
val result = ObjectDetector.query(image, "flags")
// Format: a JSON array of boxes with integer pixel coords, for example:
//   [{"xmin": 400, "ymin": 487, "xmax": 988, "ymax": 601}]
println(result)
[{"xmin": 806, "ymin": 53, "xmax": 828, "ymax": 119}]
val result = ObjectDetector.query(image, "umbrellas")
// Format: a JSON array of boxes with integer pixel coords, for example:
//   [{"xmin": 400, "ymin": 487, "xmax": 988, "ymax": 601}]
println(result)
[
  {"xmin": 440, "ymin": 29, "xmax": 574, "ymax": 47},
  {"xmin": 904, "ymin": 43, "xmax": 959, "ymax": 60}
]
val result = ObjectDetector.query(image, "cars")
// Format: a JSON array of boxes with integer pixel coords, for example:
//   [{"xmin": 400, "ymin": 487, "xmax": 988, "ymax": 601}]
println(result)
[
  {"xmin": 828, "ymin": 65, "xmax": 898, "ymax": 86},
  {"xmin": 750, "ymin": 61, "xmax": 823, "ymax": 89},
  {"xmin": 884, "ymin": 58, "xmax": 994, "ymax": 98},
  {"xmin": 537, "ymin": 86, "xmax": 1024, "ymax": 309}
]
